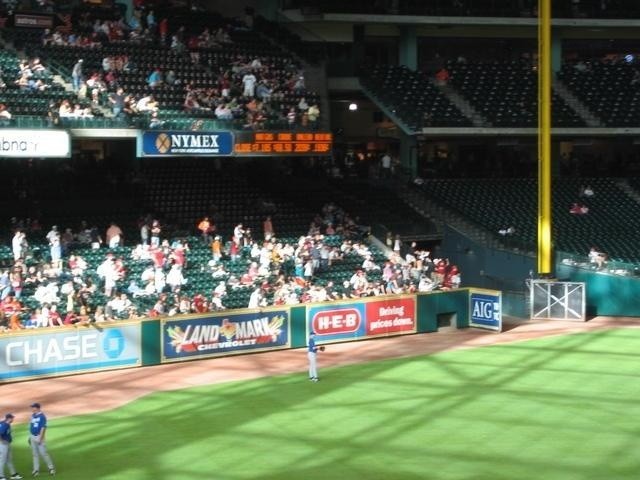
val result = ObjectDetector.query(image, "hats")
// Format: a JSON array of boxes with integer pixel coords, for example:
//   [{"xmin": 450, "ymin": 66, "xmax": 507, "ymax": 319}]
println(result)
[
  {"xmin": 6, "ymin": 413, "xmax": 14, "ymax": 417},
  {"xmin": 310, "ymin": 331, "xmax": 316, "ymax": 335},
  {"xmin": 31, "ymin": 403, "xmax": 40, "ymax": 407}
]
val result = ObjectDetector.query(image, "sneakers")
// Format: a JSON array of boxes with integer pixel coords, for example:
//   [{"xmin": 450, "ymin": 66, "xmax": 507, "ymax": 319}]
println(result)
[
  {"xmin": 1, "ymin": 477, "xmax": 7, "ymax": 480},
  {"xmin": 49, "ymin": 469, "xmax": 56, "ymax": 474},
  {"xmin": 309, "ymin": 377, "xmax": 319, "ymax": 382},
  {"xmin": 32, "ymin": 471, "xmax": 39, "ymax": 476},
  {"xmin": 10, "ymin": 473, "xmax": 24, "ymax": 480}
]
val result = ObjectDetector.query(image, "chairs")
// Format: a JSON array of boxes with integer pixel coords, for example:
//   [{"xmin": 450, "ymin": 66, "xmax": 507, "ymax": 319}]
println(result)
[{"xmin": 1, "ymin": 0, "xmax": 639, "ymax": 337}]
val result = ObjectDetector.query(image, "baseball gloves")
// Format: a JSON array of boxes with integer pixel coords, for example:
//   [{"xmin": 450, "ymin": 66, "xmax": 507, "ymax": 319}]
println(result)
[{"xmin": 319, "ymin": 347, "xmax": 325, "ymax": 351}]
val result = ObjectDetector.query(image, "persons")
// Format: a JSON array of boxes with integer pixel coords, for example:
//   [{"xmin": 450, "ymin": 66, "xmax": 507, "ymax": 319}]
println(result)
[
  {"xmin": 381, "ymin": 153, "xmax": 400, "ymax": 177},
  {"xmin": 28, "ymin": 403, "xmax": 56, "ymax": 477},
  {"xmin": 569, "ymin": 184, "xmax": 594, "ymax": 213},
  {"xmin": 1, "ymin": 1, "xmax": 320, "ymax": 130},
  {"xmin": 436, "ymin": 68, "xmax": 450, "ymax": 82},
  {"xmin": 0, "ymin": 201, "xmax": 461, "ymax": 328},
  {"xmin": 307, "ymin": 332, "xmax": 325, "ymax": 381},
  {"xmin": 588, "ymin": 247, "xmax": 608, "ymax": 270},
  {"xmin": 0, "ymin": 414, "xmax": 23, "ymax": 480},
  {"xmin": 498, "ymin": 223, "xmax": 516, "ymax": 236}
]
